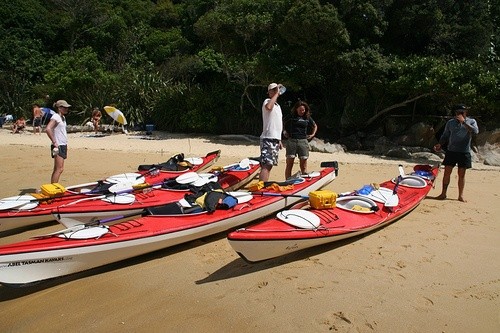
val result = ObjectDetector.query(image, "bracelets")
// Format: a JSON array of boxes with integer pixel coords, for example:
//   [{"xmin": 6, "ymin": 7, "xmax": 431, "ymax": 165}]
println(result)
[
  {"xmin": 276, "ymin": 92, "xmax": 279, "ymax": 96},
  {"xmin": 462, "ymin": 122, "xmax": 466, "ymax": 125},
  {"xmin": 53, "ymin": 141, "xmax": 56, "ymax": 143}
]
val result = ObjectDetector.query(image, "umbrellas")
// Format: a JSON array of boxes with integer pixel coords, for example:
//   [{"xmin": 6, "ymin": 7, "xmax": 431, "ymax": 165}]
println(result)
[
  {"xmin": 104, "ymin": 105, "xmax": 127, "ymax": 126},
  {"xmin": 40, "ymin": 108, "xmax": 56, "ymax": 132}
]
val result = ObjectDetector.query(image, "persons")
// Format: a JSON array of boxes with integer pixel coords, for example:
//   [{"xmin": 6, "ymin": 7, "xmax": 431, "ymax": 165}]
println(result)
[
  {"xmin": 45, "ymin": 100, "xmax": 71, "ymax": 183},
  {"xmin": 259, "ymin": 83, "xmax": 283, "ymax": 182},
  {"xmin": 92, "ymin": 108, "xmax": 102, "ymax": 134},
  {"xmin": 11, "ymin": 116, "xmax": 25, "ymax": 134},
  {"xmin": 282, "ymin": 101, "xmax": 317, "ymax": 180},
  {"xmin": 434, "ymin": 105, "xmax": 479, "ymax": 202},
  {"xmin": 32, "ymin": 104, "xmax": 43, "ymax": 134}
]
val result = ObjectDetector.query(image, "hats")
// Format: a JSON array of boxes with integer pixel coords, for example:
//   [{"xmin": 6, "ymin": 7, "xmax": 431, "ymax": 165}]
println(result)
[
  {"xmin": 56, "ymin": 99, "xmax": 72, "ymax": 107},
  {"xmin": 267, "ymin": 82, "xmax": 277, "ymax": 90},
  {"xmin": 454, "ymin": 103, "xmax": 467, "ymax": 112}
]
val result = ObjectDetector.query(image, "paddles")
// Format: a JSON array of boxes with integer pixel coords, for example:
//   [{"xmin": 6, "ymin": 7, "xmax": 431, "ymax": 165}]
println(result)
[
  {"xmin": 226, "ymin": 191, "xmax": 310, "ymax": 200},
  {"xmin": 193, "ymin": 158, "xmax": 250, "ymax": 186},
  {"xmin": 0, "ymin": 183, "xmax": 136, "ymax": 212},
  {"xmin": 29, "ymin": 195, "xmax": 190, "ymax": 238},
  {"xmin": 107, "ymin": 171, "xmax": 198, "ymax": 199},
  {"xmin": 106, "ymin": 166, "xmax": 160, "ymax": 184},
  {"xmin": 382, "ymin": 160, "xmax": 404, "ymax": 209}
]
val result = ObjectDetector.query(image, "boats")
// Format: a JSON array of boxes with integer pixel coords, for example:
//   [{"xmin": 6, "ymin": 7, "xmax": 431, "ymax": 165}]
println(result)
[
  {"xmin": 0, "ymin": 147, "xmax": 223, "ymax": 235},
  {"xmin": 0, "ymin": 158, "xmax": 343, "ymax": 289},
  {"xmin": 222, "ymin": 160, "xmax": 444, "ymax": 266},
  {"xmin": 48, "ymin": 155, "xmax": 267, "ymax": 233}
]
